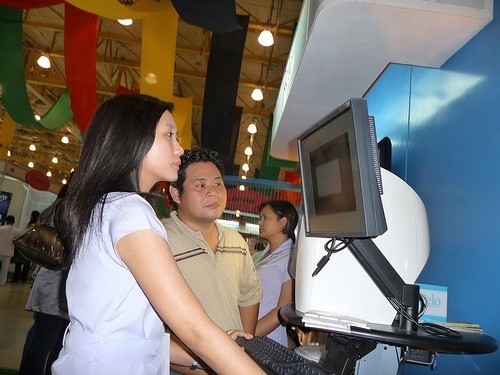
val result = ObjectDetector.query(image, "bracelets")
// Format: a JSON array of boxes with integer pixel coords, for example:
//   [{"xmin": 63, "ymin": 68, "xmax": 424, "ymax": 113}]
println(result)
[{"xmin": 190, "ymin": 355, "xmax": 198, "ymax": 370}]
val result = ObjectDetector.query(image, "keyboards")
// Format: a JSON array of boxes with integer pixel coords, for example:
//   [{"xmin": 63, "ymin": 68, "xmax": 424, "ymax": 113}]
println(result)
[{"xmin": 235, "ymin": 335, "xmax": 335, "ymax": 375}]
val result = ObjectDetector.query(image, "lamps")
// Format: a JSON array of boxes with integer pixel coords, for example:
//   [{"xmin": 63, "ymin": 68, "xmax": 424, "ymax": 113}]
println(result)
[
  {"xmin": 244, "ymin": 145, "xmax": 253, "ymax": 156},
  {"xmin": 251, "ymin": 84, "xmax": 264, "ymax": 102},
  {"xmin": 247, "ymin": 119, "xmax": 258, "ymax": 134},
  {"xmin": 257, "ymin": 22, "xmax": 274, "ymax": 47},
  {"xmin": 37, "ymin": 52, "xmax": 52, "ymax": 69}
]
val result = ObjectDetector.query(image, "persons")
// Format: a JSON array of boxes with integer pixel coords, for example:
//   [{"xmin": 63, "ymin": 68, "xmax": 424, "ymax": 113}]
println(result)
[
  {"xmin": 0, "ymin": 209, "xmax": 42, "ymax": 289},
  {"xmin": 160, "ymin": 149, "xmax": 262, "ymax": 375},
  {"xmin": 252, "ymin": 199, "xmax": 298, "ymax": 349},
  {"xmin": 50, "ymin": 93, "xmax": 269, "ymax": 375},
  {"xmin": 18, "ymin": 171, "xmax": 72, "ymax": 375}
]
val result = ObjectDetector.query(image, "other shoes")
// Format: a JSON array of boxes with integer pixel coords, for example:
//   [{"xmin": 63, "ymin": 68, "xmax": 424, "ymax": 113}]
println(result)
[{"xmin": 8, "ymin": 279, "xmax": 17, "ymax": 282}]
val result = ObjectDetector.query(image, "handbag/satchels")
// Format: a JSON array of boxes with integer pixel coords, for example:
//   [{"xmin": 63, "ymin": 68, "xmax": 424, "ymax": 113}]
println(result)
[{"xmin": 13, "ymin": 199, "xmax": 65, "ymax": 271}]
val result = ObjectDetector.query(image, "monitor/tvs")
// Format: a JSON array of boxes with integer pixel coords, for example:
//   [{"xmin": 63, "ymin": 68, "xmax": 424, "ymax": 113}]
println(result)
[{"xmin": 296, "ymin": 97, "xmax": 388, "ymax": 237}]
